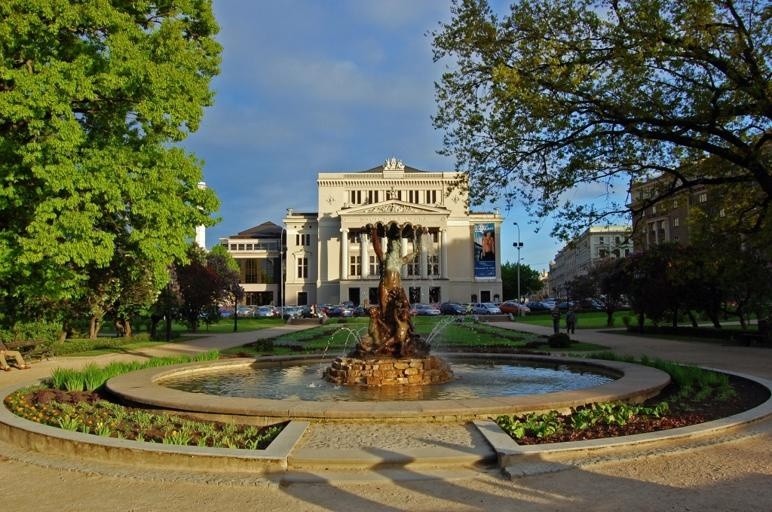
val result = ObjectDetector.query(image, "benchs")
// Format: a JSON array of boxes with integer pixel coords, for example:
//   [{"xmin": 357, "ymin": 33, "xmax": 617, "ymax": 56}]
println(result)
[{"xmin": 4, "ymin": 338, "xmax": 50, "ymax": 366}]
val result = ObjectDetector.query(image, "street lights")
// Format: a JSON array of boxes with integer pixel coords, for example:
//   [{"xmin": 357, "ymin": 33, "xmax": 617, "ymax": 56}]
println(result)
[
  {"xmin": 279, "ymin": 225, "xmax": 293, "ymax": 318},
  {"xmin": 513, "ymin": 221, "xmax": 523, "ymax": 298}
]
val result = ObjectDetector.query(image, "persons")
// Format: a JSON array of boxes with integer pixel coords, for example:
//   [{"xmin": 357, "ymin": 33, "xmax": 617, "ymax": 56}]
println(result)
[
  {"xmin": 364, "ymin": 299, "xmax": 390, "ymax": 347},
  {"xmin": 0, "ymin": 337, "xmax": 31, "ymax": 371},
  {"xmin": 482, "ymin": 230, "xmax": 495, "ymax": 262},
  {"xmin": 551, "ymin": 303, "xmax": 562, "ymax": 334},
  {"xmin": 373, "ymin": 307, "xmax": 420, "ymax": 357},
  {"xmin": 566, "ymin": 308, "xmax": 576, "ymax": 334},
  {"xmin": 367, "ymin": 223, "xmax": 423, "ymax": 321}
]
